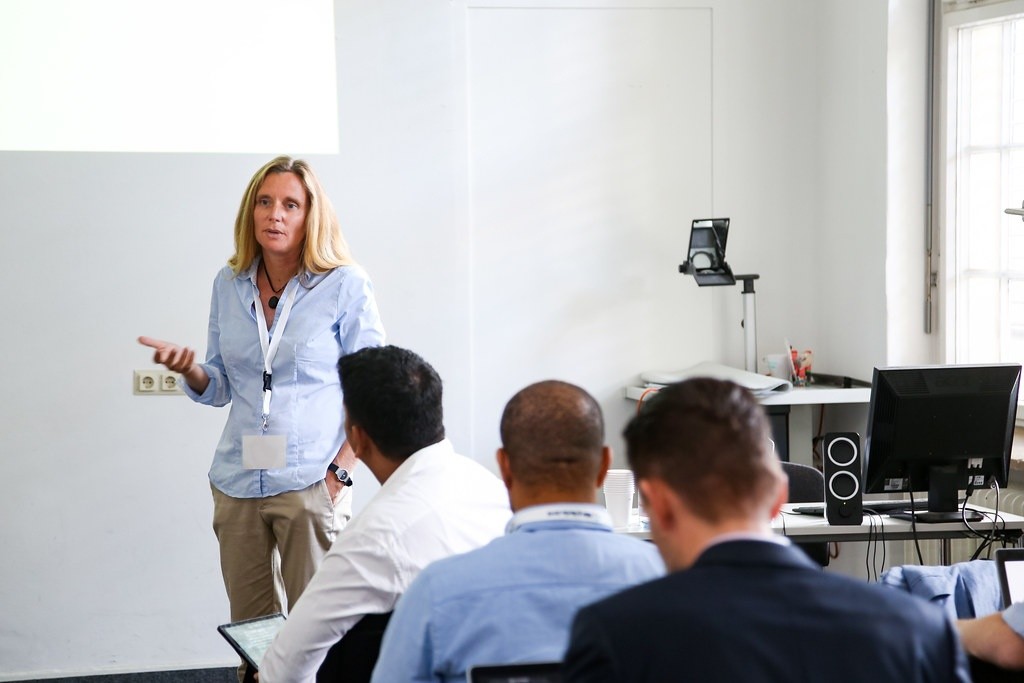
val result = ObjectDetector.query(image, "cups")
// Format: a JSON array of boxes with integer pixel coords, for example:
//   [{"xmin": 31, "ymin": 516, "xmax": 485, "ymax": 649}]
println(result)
[
  {"xmin": 602, "ymin": 468, "xmax": 636, "ymax": 528},
  {"xmin": 767, "ymin": 354, "xmax": 791, "ymax": 381}
]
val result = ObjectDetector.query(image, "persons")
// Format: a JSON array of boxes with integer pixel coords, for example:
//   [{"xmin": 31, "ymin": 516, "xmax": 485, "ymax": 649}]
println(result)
[
  {"xmin": 371, "ymin": 380, "xmax": 666, "ymax": 683},
  {"xmin": 138, "ymin": 156, "xmax": 386, "ymax": 683},
  {"xmin": 259, "ymin": 347, "xmax": 515, "ymax": 683},
  {"xmin": 560, "ymin": 375, "xmax": 957, "ymax": 683},
  {"xmin": 955, "ymin": 600, "xmax": 1024, "ymax": 668}
]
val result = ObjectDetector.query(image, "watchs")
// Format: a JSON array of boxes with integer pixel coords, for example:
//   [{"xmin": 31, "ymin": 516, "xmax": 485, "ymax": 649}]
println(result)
[{"xmin": 328, "ymin": 463, "xmax": 353, "ymax": 486}]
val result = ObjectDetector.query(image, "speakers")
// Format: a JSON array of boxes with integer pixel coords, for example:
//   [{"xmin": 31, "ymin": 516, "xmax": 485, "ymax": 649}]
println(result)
[{"xmin": 821, "ymin": 432, "xmax": 863, "ymax": 525}]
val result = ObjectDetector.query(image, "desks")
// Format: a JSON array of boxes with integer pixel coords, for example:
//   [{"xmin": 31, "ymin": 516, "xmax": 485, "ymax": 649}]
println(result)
[
  {"xmin": 604, "ymin": 502, "xmax": 1024, "ymax": 573},
  {"xmin": 629, "ymin": 367, "xmax": 874, "ymax": 469}
]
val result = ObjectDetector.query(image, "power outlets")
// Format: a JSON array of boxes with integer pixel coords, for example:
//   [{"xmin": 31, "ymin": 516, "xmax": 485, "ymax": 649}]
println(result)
[{"xmin": 132, "ymin": 367, "xmax": 186, "ymax": 395}]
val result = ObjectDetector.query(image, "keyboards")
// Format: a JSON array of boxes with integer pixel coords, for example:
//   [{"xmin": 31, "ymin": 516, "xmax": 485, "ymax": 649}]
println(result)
[{"xmin": 792, "ymin": 497, "xmax": 966, "ymax": 518}]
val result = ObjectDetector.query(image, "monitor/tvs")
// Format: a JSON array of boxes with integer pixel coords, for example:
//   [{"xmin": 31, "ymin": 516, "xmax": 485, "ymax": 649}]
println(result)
[{"xmin": 859, "ymin": 360, "xmax": 1022, "ymax": 524}]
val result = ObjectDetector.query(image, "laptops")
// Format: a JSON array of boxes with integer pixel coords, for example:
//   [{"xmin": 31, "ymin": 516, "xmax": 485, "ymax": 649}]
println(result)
[
  {"xmin": 994, "ymin": 548, "xmax": 1024, "ymax": 610},
  {"xmin": 218, "ymin": 612, "xmax": 287, "ymax": 672}
]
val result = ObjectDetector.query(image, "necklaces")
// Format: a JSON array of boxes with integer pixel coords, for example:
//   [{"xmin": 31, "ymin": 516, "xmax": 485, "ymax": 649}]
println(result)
[{"xmin": 261, "ymin": 252, "xmax": 287, "ymax": 309}]
